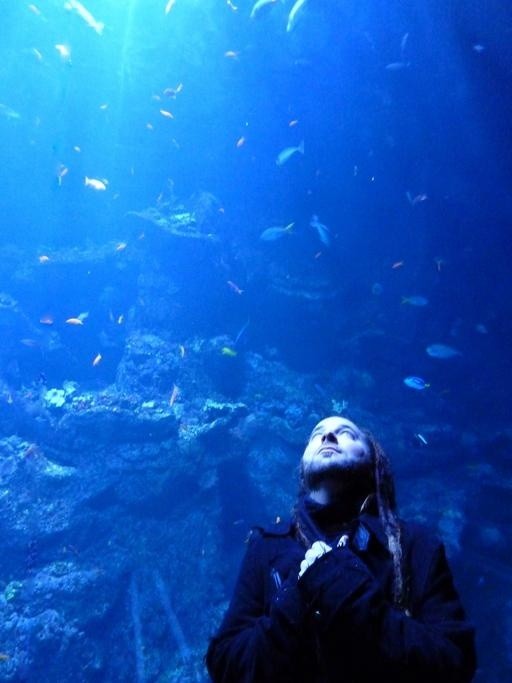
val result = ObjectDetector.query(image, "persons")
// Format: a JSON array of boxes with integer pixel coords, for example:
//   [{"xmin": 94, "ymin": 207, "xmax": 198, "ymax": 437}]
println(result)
[{"xmin": 201, "ymin": 416, "xmax": 479, "ymax": 682}]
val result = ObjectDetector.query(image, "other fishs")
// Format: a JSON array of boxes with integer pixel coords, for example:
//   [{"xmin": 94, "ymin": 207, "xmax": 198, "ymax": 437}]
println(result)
[{"xmin": 0, "ymin": 0, "xmax": 486, "ymax": 459}]
